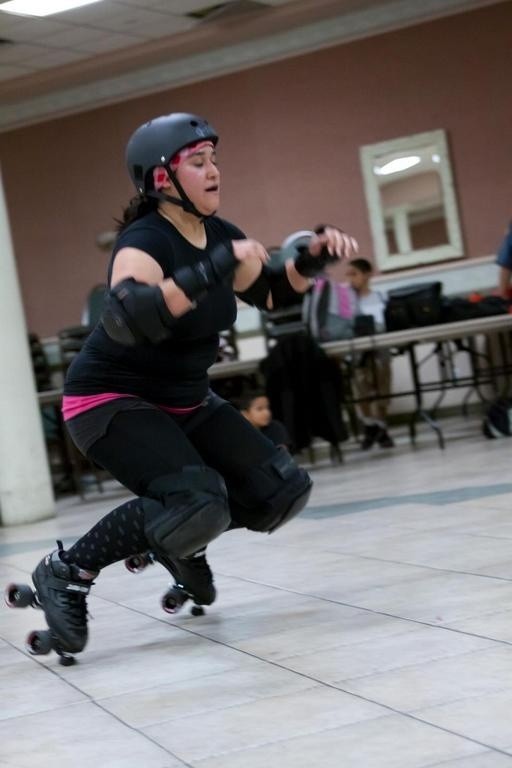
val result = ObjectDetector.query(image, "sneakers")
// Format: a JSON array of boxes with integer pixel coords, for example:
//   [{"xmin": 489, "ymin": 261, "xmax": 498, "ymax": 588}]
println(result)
[
  {"xmin": 486, "ymin": 404, "xmax": 510, "ymax": 433},
  {"xmin": 360, "ymin": 422, "xmax": 394, "ymax": 449}
]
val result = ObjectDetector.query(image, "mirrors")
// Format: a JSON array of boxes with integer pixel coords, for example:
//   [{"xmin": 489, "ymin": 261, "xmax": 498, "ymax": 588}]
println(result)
[{"xmin": 356, "ymin": 127, "xmax": 466, "ymax": 274}]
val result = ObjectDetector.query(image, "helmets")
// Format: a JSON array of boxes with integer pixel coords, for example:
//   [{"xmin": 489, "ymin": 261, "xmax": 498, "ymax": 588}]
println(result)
[{"xmin": 125, "ymin": 113, "xmax": 218, "ymax": 202}]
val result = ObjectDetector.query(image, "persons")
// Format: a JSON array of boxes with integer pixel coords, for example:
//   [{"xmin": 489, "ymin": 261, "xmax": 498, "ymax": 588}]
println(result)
[
  {"xmin": 345, "ymin": 259, "xmax": 396, "ymax": 452},
  {"xmin": 2, "ymin": 108, "xmax": 362, "ymax": 662},
  {"xmin": 476, "ymin": 223, "xmax": 511, "ymax": 440},
  {"xmin": 225, "ymin": 387, "xmax": 290, "ymax": 454},
  {"xmin": 257, "ymin": 230, "xmax": 354, "ymax": 464}
]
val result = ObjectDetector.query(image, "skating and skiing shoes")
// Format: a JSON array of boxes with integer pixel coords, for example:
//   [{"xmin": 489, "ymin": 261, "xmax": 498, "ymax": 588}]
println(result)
[
  {"xmin": 4, "ymin": 539, "xmax": 100, "ymax": 665},
  {"xmin": 125, "ymin": 544, "xmax": 216, "ymax": 615}
]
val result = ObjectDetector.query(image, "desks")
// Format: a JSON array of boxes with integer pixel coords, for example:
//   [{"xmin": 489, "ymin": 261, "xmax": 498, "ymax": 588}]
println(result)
[{"xmin": 40, "ymin": 310, "xmax": 512, "ymax": 506}]
